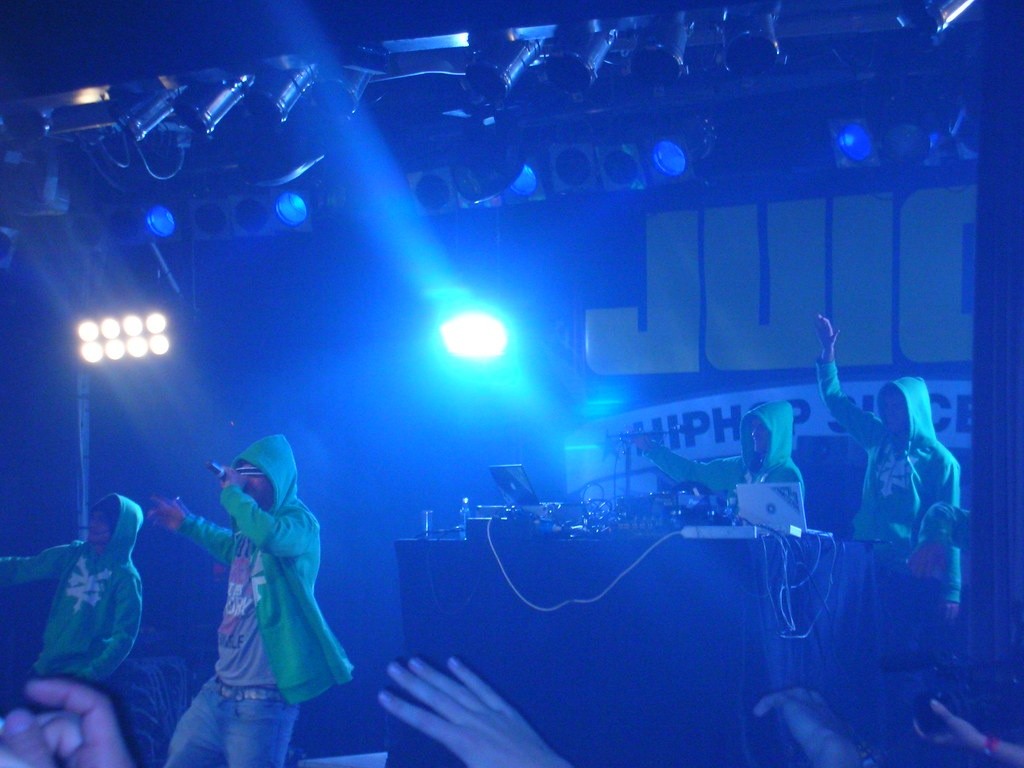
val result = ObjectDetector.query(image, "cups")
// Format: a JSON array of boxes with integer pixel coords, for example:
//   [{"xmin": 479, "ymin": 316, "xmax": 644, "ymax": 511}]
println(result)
[{"xmin": 420, "ymin": 510, "xmax": 433, "ymax": 537}]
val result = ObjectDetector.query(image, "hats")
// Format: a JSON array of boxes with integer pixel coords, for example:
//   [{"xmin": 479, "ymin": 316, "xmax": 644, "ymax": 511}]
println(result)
[{"xmin": 91, "ymin": 495, "xmax": 120, "ymax": 530}]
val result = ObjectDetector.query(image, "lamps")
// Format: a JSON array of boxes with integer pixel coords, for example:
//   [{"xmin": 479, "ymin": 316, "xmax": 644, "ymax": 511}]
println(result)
[
  {"xmin": 116, "ymin": 82, "xmax": 190, "ymax": 141},
  {"xmin": 536, "ymin": 27, "xmax": 621, "ymax": 100},
  {"xmin": 630, "ymin": 14, "xmax": 696, "ymax": 95},
  {"xmin": 192, "ymin": 67, "xmax": 263, "ymax": 137},
  {"xmin": 486, "ymin": 37, "xmax": 546, "ymax": 104},
  {"xmin": 263, "ymin": 61, "xmax": 320, "ymax": 123}
]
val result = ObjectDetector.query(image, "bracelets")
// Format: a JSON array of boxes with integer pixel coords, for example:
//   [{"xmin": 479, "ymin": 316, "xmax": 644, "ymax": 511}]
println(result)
[{"xmin": 982, "ymin": 735, "xmax": 999, "ymax": 761}]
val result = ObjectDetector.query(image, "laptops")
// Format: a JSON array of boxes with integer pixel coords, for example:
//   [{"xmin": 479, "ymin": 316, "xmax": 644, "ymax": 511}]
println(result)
[
  {"xmin": 735, "ymin": 480, "xmax": 834, "ymax": 538},
  {"xmin": 488, "ymin": 463, "xmax": 565, "ymax": 505}
]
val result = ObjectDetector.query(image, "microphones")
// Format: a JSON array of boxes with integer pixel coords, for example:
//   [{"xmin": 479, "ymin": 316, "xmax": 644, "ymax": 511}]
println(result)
[{"xmin": 207, "ymin": 462, "xmax": 227, "ymax": 482}]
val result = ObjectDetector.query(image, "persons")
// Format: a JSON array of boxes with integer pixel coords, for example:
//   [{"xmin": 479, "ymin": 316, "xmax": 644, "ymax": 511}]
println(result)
[
  {"xmin": 631, "ymin": 400, "xmax": 806, "ymax": 525},
  {"xmin": 911, "ymin": 698, "xmax": 1024, "ymax": 768},
  {"xmin": 0, "ymin": 493, "xmax": 144, "ymax": 714},
  {"xmin": 814, "ymin": 313, "xmax": 972, "ymax": 621},
  {"xmin": 376, "ymin": 655, "xmax": 573, "ymax": 768},
  {"xmin": 147, "ymin": 433, "xmax": 321, "ymax": 768},
  {"xmin": 0, "ymin": 675, "xmax": 135, "ymax": 768},
  {"xmin": 753, "ymin": 686, "xmax": 861, "ymax": 768}
]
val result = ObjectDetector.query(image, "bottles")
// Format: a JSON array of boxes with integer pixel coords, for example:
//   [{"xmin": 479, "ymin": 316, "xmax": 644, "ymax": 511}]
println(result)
[{"xmin": 460, "ymin": 497, "xmax": 469, "ymax": 526}]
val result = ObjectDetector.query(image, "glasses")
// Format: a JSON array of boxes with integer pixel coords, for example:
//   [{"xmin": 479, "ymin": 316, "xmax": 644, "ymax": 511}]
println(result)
[{"xmin": 751, "ymin": 423, "xmax": 766, "ymax": 432}]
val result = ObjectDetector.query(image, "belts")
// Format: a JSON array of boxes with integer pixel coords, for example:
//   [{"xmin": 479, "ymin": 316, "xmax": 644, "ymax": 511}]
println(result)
[{"xmin": 215, "ymin": 678, "xmax": 286, "ymax": 701}]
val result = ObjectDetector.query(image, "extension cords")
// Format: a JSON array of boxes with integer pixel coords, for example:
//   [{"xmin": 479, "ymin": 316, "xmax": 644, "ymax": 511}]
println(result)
[{"xmin": 678, "ymin": 526, "xmax": 757, "ymax": 540}]
